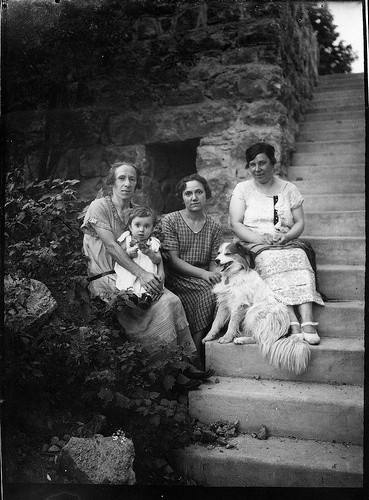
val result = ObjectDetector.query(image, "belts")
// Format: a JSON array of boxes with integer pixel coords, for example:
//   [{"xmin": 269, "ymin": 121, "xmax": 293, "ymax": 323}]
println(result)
[{"xmin": 86, "ymin": 270, "xmax": 116, "ymax": 283}]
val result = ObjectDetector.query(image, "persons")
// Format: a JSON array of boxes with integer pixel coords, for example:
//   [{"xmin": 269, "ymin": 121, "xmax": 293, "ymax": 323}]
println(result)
[
  {"xmin": 162, "ymin": 174, "xmax": 224, "ymax": 355},
  {"xmin": 80, "ymin": 162, "xmax": 215, "ymax": 391},
  {"xmin": 228, "ymin": 142, "xmax": 325, "ymax": 345},
  {"xmin": 114, "ymin": 205, "xmax": 162, "ymax": 308}
]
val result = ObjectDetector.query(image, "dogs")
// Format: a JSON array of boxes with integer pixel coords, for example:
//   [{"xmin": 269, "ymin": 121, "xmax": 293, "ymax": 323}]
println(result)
[{"xmin": 201, "ymin": 240, "xmax": 310, "ymax": 376}]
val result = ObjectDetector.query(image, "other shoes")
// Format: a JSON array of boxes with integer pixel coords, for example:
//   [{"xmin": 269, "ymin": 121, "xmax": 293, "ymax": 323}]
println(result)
[
  {"xmin": 189, "ymin": 366, "xmax": 215, "ymax": 380},
  {"xmin": 290, "ymin": 322, "xmax": 302, "ymax": 341},
  {"xmin": 177, "ymin": 378, "xmax": 201, "ymax": 391},
  {"xmin": 301, "ymin": 321, "xmax": 320, "ymax": 344}
]
